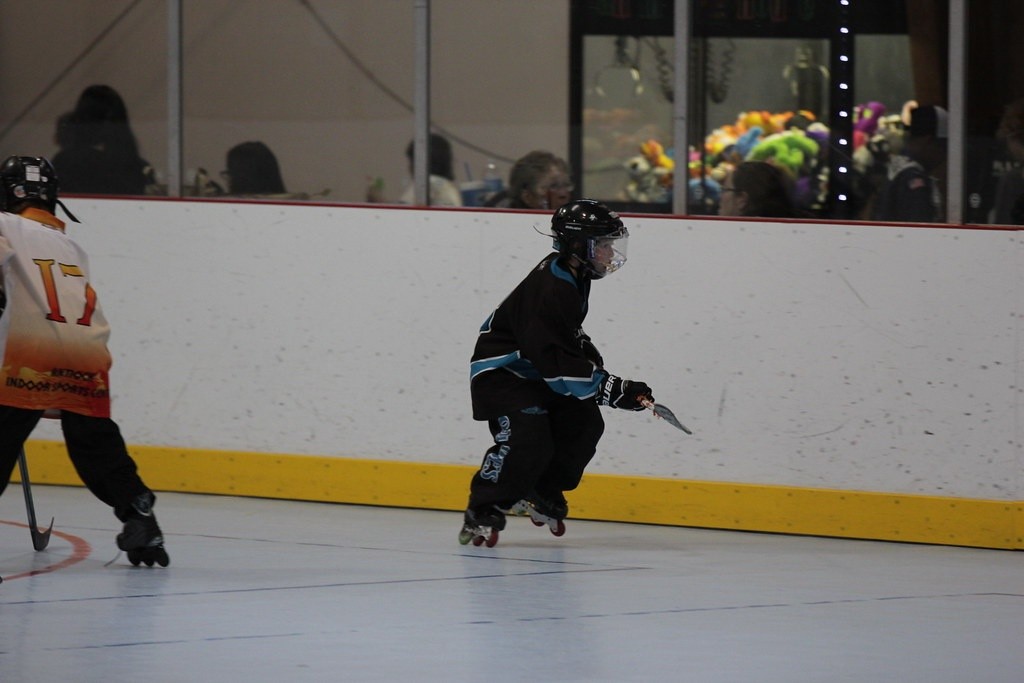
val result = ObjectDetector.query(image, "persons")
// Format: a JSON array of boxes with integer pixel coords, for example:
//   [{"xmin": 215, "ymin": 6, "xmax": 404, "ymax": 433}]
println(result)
[
  {"xmin": 44, "ymin": 84, "xmax": 570, "ymax": 208},
  {"xmin": 0, "ymin": 154, "xmax": 170, "ymax": 569},
  {"xmin": 721, "ymin": 103, "xmax": 1023, "ymax": 227},
  {"xmin": 455, "ymin": 201, "xmax": 656, "ymax": 545}
]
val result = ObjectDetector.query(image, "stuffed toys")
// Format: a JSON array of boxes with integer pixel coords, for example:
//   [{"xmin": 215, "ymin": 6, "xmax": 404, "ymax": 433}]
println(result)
[{"xmin": 624, "ymin": 104, "xmax": 908, "ymax": 209}]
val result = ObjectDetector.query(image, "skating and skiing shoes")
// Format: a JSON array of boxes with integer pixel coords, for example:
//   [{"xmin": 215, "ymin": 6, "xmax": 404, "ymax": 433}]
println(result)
[
  {"xmin": 508, "ymin": 481, "xmax": 568, "ymax": 535},
  {"xmin": 116, "ymin": 493, "xmax": 170, "ymax": 569},
  {"xmin": 458, "ymin": 497, "xmax": 506, "ymax": 548}
]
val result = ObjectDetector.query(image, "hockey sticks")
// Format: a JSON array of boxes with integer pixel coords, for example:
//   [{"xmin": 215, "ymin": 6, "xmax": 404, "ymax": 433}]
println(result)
[
  {"xmin": 17, "ymin": 442, "xmax": 55, "ymax": 554},
  {"xmin": 635, "ymin": 392, "xmax": 695, "ymax": 436}
]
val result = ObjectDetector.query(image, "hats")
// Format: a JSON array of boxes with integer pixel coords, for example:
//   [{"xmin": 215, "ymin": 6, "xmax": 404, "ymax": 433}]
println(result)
[{"xmin": 907, "ymin": 105, "xmax": 949, "ymax": 139}]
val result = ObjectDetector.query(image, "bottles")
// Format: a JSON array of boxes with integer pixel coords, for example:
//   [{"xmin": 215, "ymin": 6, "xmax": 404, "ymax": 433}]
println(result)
[{"xmin": 484, "ymin": 163, "xmax": 500, "ymax": 201}]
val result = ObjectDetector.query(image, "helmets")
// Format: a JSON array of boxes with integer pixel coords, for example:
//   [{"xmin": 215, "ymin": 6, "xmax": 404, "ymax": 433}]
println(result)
[
  {"xmin": 552, "ymin": 199, "xmax": 629, "ymax": 280},
  {"xmin": 0, "ymin": 155, "xmax": 60, "ymax": 217}
]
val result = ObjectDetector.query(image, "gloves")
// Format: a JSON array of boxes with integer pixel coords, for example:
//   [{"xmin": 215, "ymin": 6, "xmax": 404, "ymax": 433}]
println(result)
[
  {"xmin": 597, "ymin": 374, "xmax": 655, "ymax": 411},
  {"xmin": 575, "ymin": 326, "xmax": 604, "ymax": 367}
]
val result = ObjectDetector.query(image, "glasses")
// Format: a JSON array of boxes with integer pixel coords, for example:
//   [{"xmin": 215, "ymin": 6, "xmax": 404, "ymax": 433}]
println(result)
[
  {"xmin": 536, "ymin": 180, "xmax": 575, "ymax": 193},
  {"xmin": 717, "ymin": 185, "xmax": 749, "ymax": 194}
]
